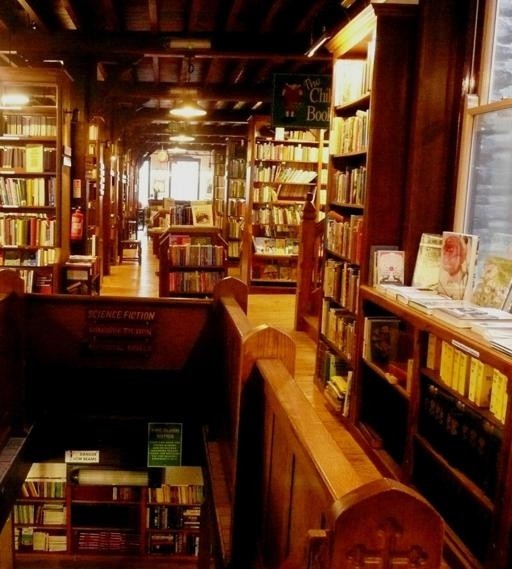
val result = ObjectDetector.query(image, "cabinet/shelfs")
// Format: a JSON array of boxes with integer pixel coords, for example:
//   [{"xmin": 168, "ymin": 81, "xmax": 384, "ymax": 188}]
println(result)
[
  {"xmin": 11, "ymin": 462, "xmax": 201, "ymax": 568},
  {"xmin": 209, "ymin": 116, "xmax": 329, "ymax": 293},
  {"xmin": 0, "ymin": 64, "xmax": 139, "ymax": 295},
  {"xmin": 350, "ymin": 283, "xmax": 512, "ymax": 569},
  {"xmin": 313, "ymin": 5, "xmax": 415, "ymax": 430},
  {"xmin": 146, "ymin": 194, "xmax": 230, "ymax": 299}
]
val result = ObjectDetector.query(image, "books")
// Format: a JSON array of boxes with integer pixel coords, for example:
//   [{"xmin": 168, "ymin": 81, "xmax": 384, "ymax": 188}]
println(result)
[
  {"xmin": 252, "ymin": 141, "xmax": 329, "ymax": 282},
  {"xmin": 113, "ymin": 485, "xmax": 132, "ymax": 500},
  {"xmin": 170, "ymin": 205, "xmax": 213, "ymax": 226},
  {"xmin": 214, "ymin": 158, "xmax": 246, "ymax": 257},
  {"xmin": 321, "ymin": 60, "xmax": 373, "ymax": 419},
  {"xmin": 0, "ymin": 115, "xmax": 56, "ymax": 293},
  {"xmin": 13, "ymin": 481, "xmax": 67, "ymax": 552},
  {"xmin": 147, "ymin": 484, "xmax": 201, "ymax": 557},
  {"xmin": 361, "ymin": 231, "xmax": 512, "ymax": 499},
  {"xmin": 167, "ymin": 234, "xmax": 224, "ymax": 294},
  {"xmin": 78, "ymin": 531, "xmax": 139, "ymax": 551}
]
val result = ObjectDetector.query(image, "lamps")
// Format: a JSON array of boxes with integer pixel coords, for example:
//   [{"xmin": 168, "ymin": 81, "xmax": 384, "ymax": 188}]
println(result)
[{"xmin": 167, "ymin": 54, "xmax": 208, "ymax": 156}]
